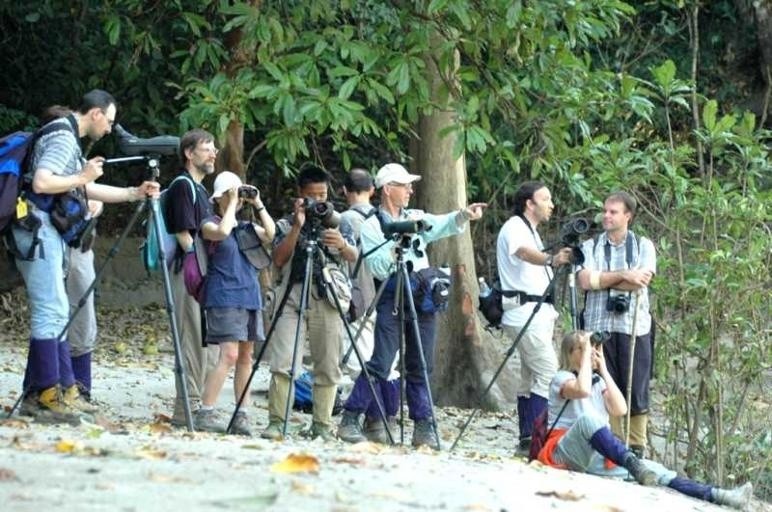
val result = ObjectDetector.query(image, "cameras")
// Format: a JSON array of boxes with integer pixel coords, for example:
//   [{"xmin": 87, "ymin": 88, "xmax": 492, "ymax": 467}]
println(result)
[
  {"xmin": 606, "ymin": 294, "xmax": 630, "ymax": 313},
  {"xmin": 304, "ymin": 196, "xmax": 334, "ymax": 223}
]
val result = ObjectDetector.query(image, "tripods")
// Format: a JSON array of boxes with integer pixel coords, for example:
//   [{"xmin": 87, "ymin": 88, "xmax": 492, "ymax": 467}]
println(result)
[
  {"xmin": 6, "ymin": 160, "xmax": 195, "ymax": 432},
  {"xmin": 226, "ymin": 238, "xmax": 396, "ymax": 446},
  {"xmin": 343, "ymin": 249, "xmax": 440, "ymax": 451},
  {"xmin": 449, "ymin": 242, "xmax": 581, "ymax": 459}
]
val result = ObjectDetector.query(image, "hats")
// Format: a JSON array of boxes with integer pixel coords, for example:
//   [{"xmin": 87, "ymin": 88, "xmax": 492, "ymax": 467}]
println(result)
[
  {"xmin": 208, "ymin": 169, "xmax": 244, "ymax": 205},
  {"xmin": 375, "ymin": 162, "xmax": 423, "ymax": 189}
]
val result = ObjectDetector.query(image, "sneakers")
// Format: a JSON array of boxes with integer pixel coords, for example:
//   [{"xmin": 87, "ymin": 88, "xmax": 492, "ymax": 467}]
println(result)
[
  {"xmin": 411, "ymin": 420, "xmax": 439, "ymax": 450},
  {"xmin": 386, "ymin": 417, "xmax": 401, "ymax": 442},
  {"xmin": 368, "ymin": 419, "xmax": 385, "ymax": 443},
  {"xmin": 230, "ymin": 411, "xmax": 252, "ymax": 433},
  {"xmin": 309, "ymin": 421, "xmax": 335, "ymax": 442},
  {"xmin": 26, "ymin": 389, "xmax": 81, "ymax": 420},
  {"xmin": 340, "ymin": 410, "xmax": 366, "ymax": 443},
  {"xmin": 262, "ymin": 421, "xmax": 285, "ymax": 440},
  {"xmin": 171, "ymin": 403, "xmax": 202, "ymax": 424},
  {"xmin": 63, "ymin": 385, "xmax": 101, "ymax": 413},
  {"xmin": 194, "ymin": 409, "xmax": 224, "ymax": 431}
]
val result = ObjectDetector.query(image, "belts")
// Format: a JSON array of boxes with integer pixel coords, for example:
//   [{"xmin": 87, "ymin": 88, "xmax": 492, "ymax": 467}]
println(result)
[{"xmin": 525, "ymin": 294, "xmax": 554, "ymax": 304}]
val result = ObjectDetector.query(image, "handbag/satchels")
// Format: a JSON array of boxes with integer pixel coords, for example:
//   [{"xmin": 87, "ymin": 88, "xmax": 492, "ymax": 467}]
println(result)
[
  {"xmin": 384, "ymin": 261, "xmax": 452, "ymax": 317},
  {"xmin": 27, "ymin": 184, "xmax": 97, "ymax": 254},
  {"xmin": 477, "ymin": 290, "xmax": 524, "ymax": 327},
  {"xmin": 346, "ymin": 282, "xmax": 365, "ymax": 321},
  {"xmin": 318, "ymin": 247, "xmax": 352, "ymax": 312}
]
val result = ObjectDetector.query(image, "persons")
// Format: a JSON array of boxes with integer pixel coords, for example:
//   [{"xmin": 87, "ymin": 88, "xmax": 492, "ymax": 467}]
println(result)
[
  {"xmin": 575, "ymin": 191, "xmax": 656, "ymax": 459},
  {"xmin": 261, "ymin": 166, "xmax": 359, "ymax": 443},
  {"xmin": 537, "ymin": 330, "xmax": 753, "ymax": 512},
  {"xmin": 496, "ymin": 181, "xmax": 573, "ymax": 456},
  {"xmin": 11, "ymin": 89, "xmax": 161, "ymax": 428},
  {"xmin": 194, "ymin": 171, "xmax": 276, "ymax": 436},
  {"xmin": 164, "ymin": 128, "xmax": 220, "ymax": 426},
  {"xmin": 340, "ymin": 167, "xmax": 401, "ymax": 444},
  {"xmin": 336, "ymin": 162, "xmax": 488, "ymax": 450},
  {"xmin": 38, "ymin": 105, "xmax": 104, "ymax": 403}
]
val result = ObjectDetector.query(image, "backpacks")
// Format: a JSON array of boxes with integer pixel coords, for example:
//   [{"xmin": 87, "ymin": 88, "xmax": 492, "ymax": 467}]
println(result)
[
  {"xmin": 182, "ymin": 215, "xmax": 221, "ymax": 305},
  {"xmin": 1, "ymin": 122, "xmax": 72, "ymax": 227},
  {"xmin": 529, "ymin": 395, "xmax": 568, "ymax": 461},
  {"xmin": 139, "ymin": 176, "xmax": 198, "ymax": 274}
]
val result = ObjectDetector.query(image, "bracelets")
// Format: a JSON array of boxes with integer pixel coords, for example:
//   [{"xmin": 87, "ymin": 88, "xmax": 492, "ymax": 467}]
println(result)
[
  {"xmin": 257, "ymin": 207, "xmax": 264, "ymax": 211},
  {"xmin": 461, "ymin": 208, "xmax": 468, "ymax": 221},
  {"xmin": 544, "ymin": 255, "xmax": 553, "ymax": 265},
  {"xmin": 338, "ymin": 239, "xmax": 348, "ymax": 251},
  {"xmin": 127, "ymin": 186, "xmax": 134, "ymax": 202}
]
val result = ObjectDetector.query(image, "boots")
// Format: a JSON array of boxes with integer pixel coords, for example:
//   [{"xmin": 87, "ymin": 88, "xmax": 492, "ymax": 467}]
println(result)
[
  {"xmin": 624, "ymin": 458, "xmax": 660, "ymax": 487},
  {"xmin": 716, "ymin": 482, "xmax": 753, "ymax": 511}
]
